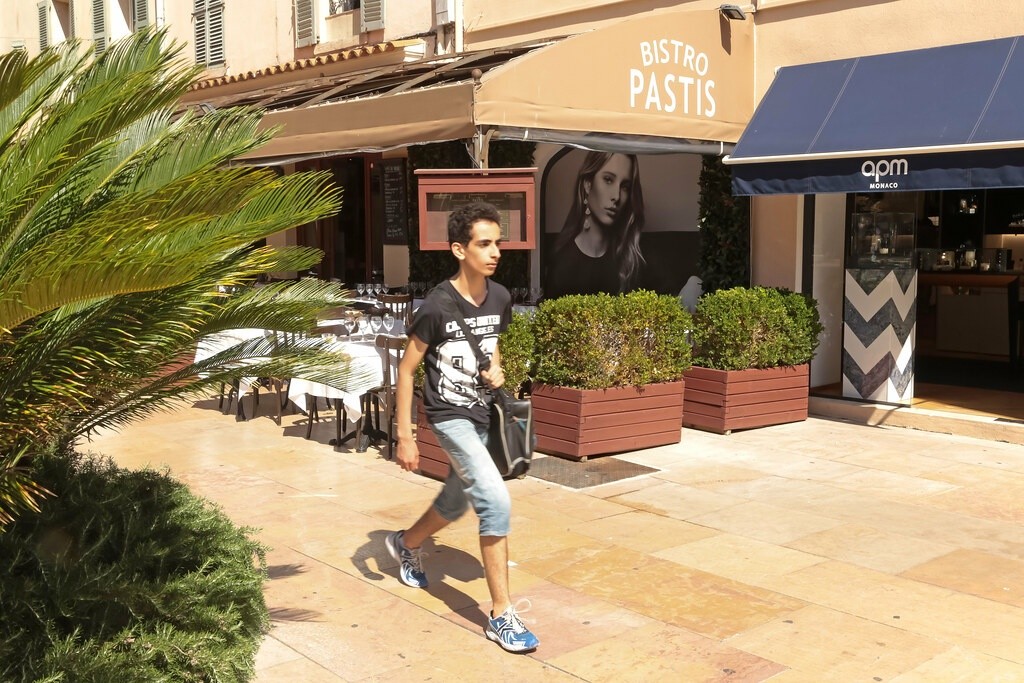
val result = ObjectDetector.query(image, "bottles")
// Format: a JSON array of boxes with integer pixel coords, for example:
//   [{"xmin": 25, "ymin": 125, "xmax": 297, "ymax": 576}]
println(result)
[{"xmin": 880, "ymin": 234, "xmax": 890, "ymax": 249}]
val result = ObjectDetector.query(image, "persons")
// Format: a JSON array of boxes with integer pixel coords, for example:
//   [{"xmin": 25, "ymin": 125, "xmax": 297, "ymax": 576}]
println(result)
[
  {"xmin": 540, "ymin": 150, "xmax": 647, "ymax": 302},
  {"xmin": 385, "ymin": 199, "xmax": 540, "ymax": 654}
]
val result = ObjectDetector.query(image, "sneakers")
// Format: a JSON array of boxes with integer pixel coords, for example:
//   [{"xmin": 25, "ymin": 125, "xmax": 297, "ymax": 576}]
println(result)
[
  {"xmin": 385, "ymin": 529, "xmax": 429, "ymax": 588},
  {"xmin": 486, "ymin": 599, "xmax": 540, "ymax": 652}
]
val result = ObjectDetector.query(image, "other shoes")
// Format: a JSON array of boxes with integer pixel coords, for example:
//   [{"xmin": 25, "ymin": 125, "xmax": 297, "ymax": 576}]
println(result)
[{"xmin": 489, "ymin": 399, "xmax": 535, "ymax": 482}]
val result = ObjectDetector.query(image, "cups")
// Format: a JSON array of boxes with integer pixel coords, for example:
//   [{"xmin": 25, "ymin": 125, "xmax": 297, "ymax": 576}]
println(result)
[{"xmin": 382, "ymin": 283, "xmax": 390, "ymax": 294}]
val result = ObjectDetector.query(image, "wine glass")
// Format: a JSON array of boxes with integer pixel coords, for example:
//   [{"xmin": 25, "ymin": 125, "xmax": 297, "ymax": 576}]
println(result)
[
  {"xmin": 343, "ymin": 318, "xmax": 355, "ymax": 343},
  {"xmin": 512, "ymin": 288, "xmax": 520, "ymax": 304},
  {"xmin": 403, "ymin": 282, "xmax": 434, "ymax": 297},
  {"xmin": 383, "ymin": 315, "xmax": 395, "ymax": 337},
  {"xmin": 357, "ymin": 283, "xmax": 365, "ymax": 300},
  {"xmin": 374, "ymin": 283, "xmax": 382, "ymax": 299},
  {"xmin": 366, "ymin": 283, "xmax": 374, "ymax": 301},
  {"xmin": 520, "ymin": 288, "xmax": 528, "ymax": 304},
  {"xmin": 370, "ymin": 315, "xmax": 382, "ymax": 341},
  {"xmin": 356, "ymin": 317, "xmax": 369, "ymax": 342}
]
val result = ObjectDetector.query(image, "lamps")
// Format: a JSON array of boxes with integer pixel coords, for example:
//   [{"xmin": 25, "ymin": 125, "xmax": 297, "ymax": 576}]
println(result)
[{"xmin": 721, "ymin": 3, "xmax": 746, "ymax": 20}]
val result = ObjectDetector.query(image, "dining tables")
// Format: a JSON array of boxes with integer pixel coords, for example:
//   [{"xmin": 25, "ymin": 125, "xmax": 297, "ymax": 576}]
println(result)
[{"xmin": 194, "ymin": 282, "xmax": 424, "ymax": 445}]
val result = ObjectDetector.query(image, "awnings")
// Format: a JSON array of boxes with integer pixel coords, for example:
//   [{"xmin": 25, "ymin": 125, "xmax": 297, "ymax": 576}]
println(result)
[
  {"xmin": 227, "ymin": 7, "xmax": 756, "ymax": 168},
  {"xmin": 721, "ymin": 34, "xmax": 1024, "ymax": 197}
]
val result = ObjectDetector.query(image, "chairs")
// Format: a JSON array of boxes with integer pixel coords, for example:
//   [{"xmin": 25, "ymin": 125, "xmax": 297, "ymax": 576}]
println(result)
[
  {"xmin": 306, "ymin": 324, "xmax": 347, "ymax": 439},
  {"xmin": 376, "ymin": 289, "xmax": 415, "ymax": 325},
  {"xmin": 368, "ymin": 307, "xmax": 390, "ymax": 321},
  {"xmin": 252, "ymin": 319, "xmax": 318, "ymax": 426},
  {"xmin": 343, "ymin": 290, "xmax": 360, "ymax": 298},
  {"xmin": 381, "ymin": 285, "xmax": 412, "ymax": 294},
  {"xmin": 321, "ymin": 307, "xmax": 353, "ymax": 319},
  {"xmin": 354, "ymin": 334, "xmax": 405, "ymax": 461}
]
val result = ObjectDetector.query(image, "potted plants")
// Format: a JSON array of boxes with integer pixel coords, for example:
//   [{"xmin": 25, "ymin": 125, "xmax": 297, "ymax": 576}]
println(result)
[
  {"xmin": 681, "ymin": 286, "xmax": 824, "ymax": 435},
  {"xmin": 533, "ymin": 287, "xmax": 694, "ymax": 462},
  {"xmin": 412, "ymin": 311, "xmax": 538, "ymax": 480}
]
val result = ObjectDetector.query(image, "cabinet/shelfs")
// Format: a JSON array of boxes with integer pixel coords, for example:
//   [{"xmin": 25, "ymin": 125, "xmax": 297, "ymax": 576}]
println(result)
[{"xmin": 850, "ymin": 212, "xmax": 915, "ymax": 254}]
